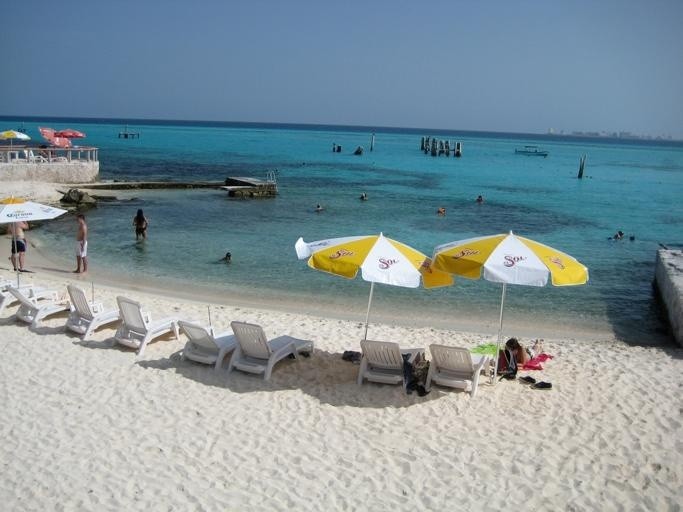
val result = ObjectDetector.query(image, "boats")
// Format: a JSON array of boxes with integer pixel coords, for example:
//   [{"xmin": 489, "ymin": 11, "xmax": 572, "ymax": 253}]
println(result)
[{"xmin": 513, "ymin": 145, "xmax": 549, "ymax": 157}]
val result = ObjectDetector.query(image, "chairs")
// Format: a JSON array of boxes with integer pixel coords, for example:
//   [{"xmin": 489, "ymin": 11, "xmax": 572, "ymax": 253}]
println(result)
[
  {"xmin": 423, "ymin": 344, "xmax": 494, "ymax": 398},
  {"xmin": 357, "ymin": 340, "xmax": 425, "ymax": 395},
  {"xmin": 0, "ymin": 149, "xmax": 68, "ymax": 163},
  {"xmin": 227, "ymin": 322, "xmax": 314, "ymax": 382},
  {"xmin": 62, "ymin": 285, "xmax": 122, "ymax": 341},
  {"xmin": 5, "ymin": 284, "xmax": 104, "ymax": 330},
  {"xmin": 177, "ymin": 320, "xmax": 237, "ymax": 374},
  {"xmin": 0, "ymin": 280, "xmax": 59, "ymax": 314},
  {"xmin": 109, "ymin": 296, "xmax": 182, "ymax": 357}
]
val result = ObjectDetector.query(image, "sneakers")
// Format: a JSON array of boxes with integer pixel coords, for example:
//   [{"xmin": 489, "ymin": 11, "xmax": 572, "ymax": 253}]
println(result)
[{"xmin": 519, "ymin": 376, "xmax": 552, "ymax": 390}]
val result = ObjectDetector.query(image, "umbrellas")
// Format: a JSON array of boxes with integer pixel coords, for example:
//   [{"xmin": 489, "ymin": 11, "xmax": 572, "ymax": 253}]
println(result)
[
  {"xmin": 295, "ymin": 231, "xmax": 455, "ymax": 358},
  {"xmin": 0, "ymin": 129, "xmax": 32, "ymax": 147},
  {"xmin": 0, "ymin": 195, "xmax": 69, "ymax": 271},
  {"xmin": 38, "ymin": 127, "xmax": 72, "ymax": 149},
  {"xmin": 54, "ymin": 128, "xmax": 87, "ymax": 148},
  {"xmin": 432, "ymin": 229, "xmax": 589, "ymax": 384}
]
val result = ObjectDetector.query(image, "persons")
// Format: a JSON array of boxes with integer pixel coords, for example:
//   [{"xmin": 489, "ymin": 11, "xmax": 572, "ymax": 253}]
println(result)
[
  {"xmin": 614, "ymin": 231, "xmax": 624, "ymax": 241},
  {"xmin": 9, "ymin": 221, "xmax": 29, "ymax": 271},
  {"xmin": 72, "ymin": 215, "xmax": 88, "ymax": 273},
  {"xmin": 315, "ymin": 204, "xmax": 323, "ymax": 211},
  {"xmin": 475, "ymin": 195, "xmax": 484, "ymax": 203},
  {"xmin": 219, "ymin": 252, "xmax": 233, "ymax": 262},
  {"xmin": 437, "ymin": 208, "xmax": 446, "ymax": 216},
  {"xmin": 132, "ymin": 209, "xmax": 148, "ymax": 240},
  {"xmin": 505, "ymin": 337, "xmax": 544, "ymax": 367}
]
